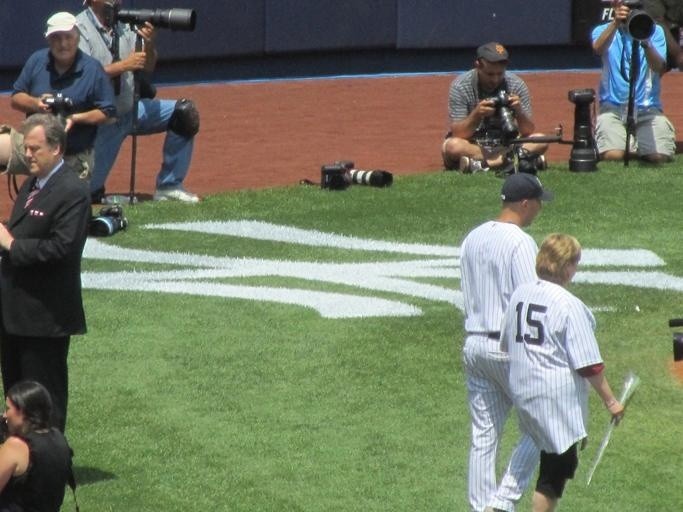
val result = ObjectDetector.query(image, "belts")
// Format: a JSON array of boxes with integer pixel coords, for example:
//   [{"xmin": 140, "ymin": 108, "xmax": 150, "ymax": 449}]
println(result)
[{"xmin": 467, "ymin": 331, "xmax": 501, "ymax": 340}]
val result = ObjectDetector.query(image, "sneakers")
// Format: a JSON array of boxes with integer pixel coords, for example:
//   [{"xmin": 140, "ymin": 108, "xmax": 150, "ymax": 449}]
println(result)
[
  {"xmin": 153, "ymin": 188, "xmax": 202, "ymax": 205},
  {"xmin": 527, "ymin": 155, "xmax": 548, "ymax": 171},
  {"xmin": 459, "ymin": 155, "xmax": 490, "ymax": 175}
]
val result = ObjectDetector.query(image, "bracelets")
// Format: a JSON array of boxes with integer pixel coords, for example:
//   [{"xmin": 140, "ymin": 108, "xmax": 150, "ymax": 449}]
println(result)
[{"xmin": 606, "ymin": 398, "xmax": 616, "ymax": 408}]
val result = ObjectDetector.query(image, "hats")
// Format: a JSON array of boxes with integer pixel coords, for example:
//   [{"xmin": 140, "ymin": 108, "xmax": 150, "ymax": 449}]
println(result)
[
  {"xmin": 474, "ymin": 42, "xmax": 512, "ymax": 64},
  {"xmin": 44, "ymin": 12, "xmax": 78, "ymax": 39},
  {"xmin": 500, "ymin": 173, "xmax": 553, "ymax": 203}
]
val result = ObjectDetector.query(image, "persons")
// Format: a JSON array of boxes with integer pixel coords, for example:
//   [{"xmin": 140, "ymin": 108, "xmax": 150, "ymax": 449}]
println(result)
[
  {"xmin": 634, "ymin": 0, "xmax": 682, "ymax": 73},
  {"xmin": 592, "ymin": 0, "xmax": 676, "ymax": 164},
  {"xmin": 500, "ymin": 233, "xmax": 625, "ymax": 512},
  {"xmin": 75, "ymin": 0, "xmax": 201, "ymax": 204},
  {"xmin": 460, "ymin": 172, "xmax": 555, "ymax": 512},
  {"xmin": 0, "ymin": 112, "xmax": 92, "ymax": 435},
  {"xmin": 0, "ymin": 380, "xmax": 71, "ymax": 512},
  {"xmin": 0, "ymin": 12, "xmax": 118, "ymax": 178},
  {"xmin": 442, "ymin": 42, "xmax": 549, "ymax": 173}
]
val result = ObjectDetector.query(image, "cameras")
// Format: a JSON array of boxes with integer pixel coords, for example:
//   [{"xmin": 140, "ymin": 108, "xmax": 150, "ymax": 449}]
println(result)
[
  {"xmin": 41, "ymin": 91, "xmax": 76, "ymax": 132},
  {"xmin": 320, "ymin": 158, "xmax": 394, "ymax": 187},
  {"xmin": 486, "ymin": 89, "xmax": 523, "ymax": 141}
]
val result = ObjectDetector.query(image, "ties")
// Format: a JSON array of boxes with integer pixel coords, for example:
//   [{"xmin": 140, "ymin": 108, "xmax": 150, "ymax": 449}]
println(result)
[{"xmin": 24, "ymin": 186, "xmax": 42, "ymax": 210}]
